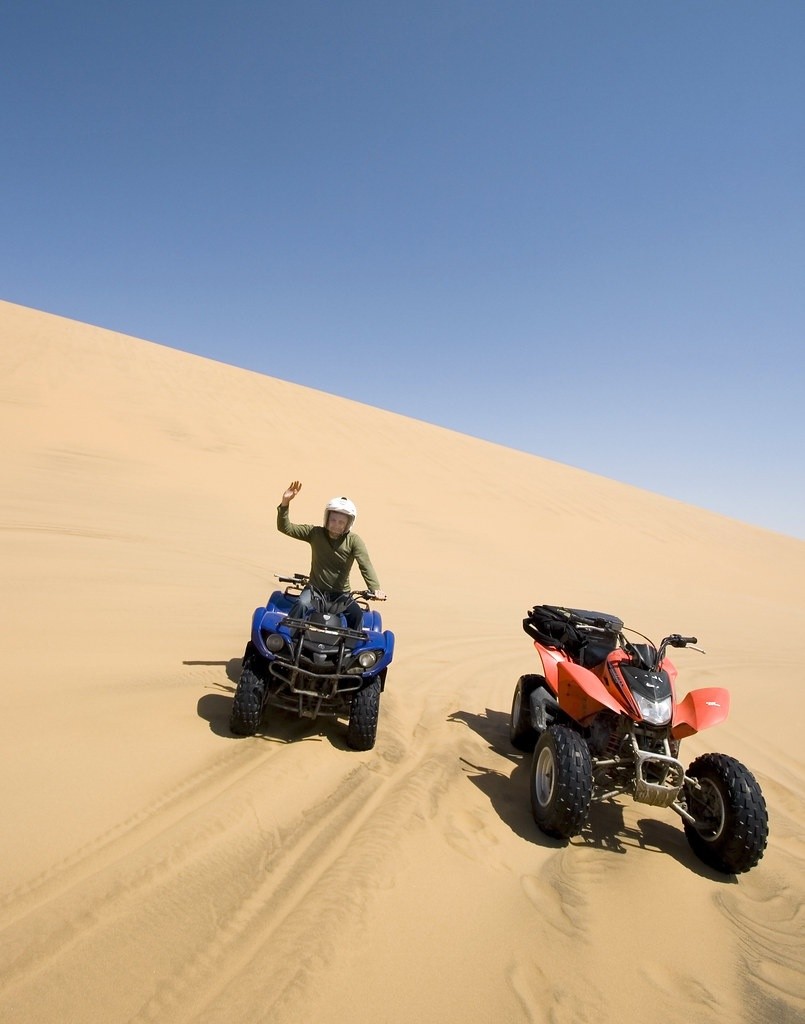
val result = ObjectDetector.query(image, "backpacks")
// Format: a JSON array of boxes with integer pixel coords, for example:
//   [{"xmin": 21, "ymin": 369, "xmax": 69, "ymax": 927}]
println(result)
[{"xmin": 522, "ymin": 605, "xmax": 626, "ymax": 669}]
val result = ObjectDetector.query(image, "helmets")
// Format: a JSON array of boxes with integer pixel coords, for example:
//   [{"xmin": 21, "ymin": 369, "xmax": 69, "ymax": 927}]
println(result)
[{"xmin": 322, "ymin": 496, "xmax": 356, "ymax": 535}]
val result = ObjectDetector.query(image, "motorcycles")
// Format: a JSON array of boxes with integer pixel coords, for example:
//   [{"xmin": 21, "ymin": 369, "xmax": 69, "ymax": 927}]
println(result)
[
  {"xmin": 509, "ymin": 604, "xmax": 770, "ymax": 876},
  {"xmin": 230, "ymin": 574, "xmax": 394, "ymax": 750}
]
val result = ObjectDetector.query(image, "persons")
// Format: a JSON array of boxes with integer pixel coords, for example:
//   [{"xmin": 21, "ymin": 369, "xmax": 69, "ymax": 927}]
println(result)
[{"xmin": 277, "ymin": 480, "xmax": 386, "ymax": 657}]
